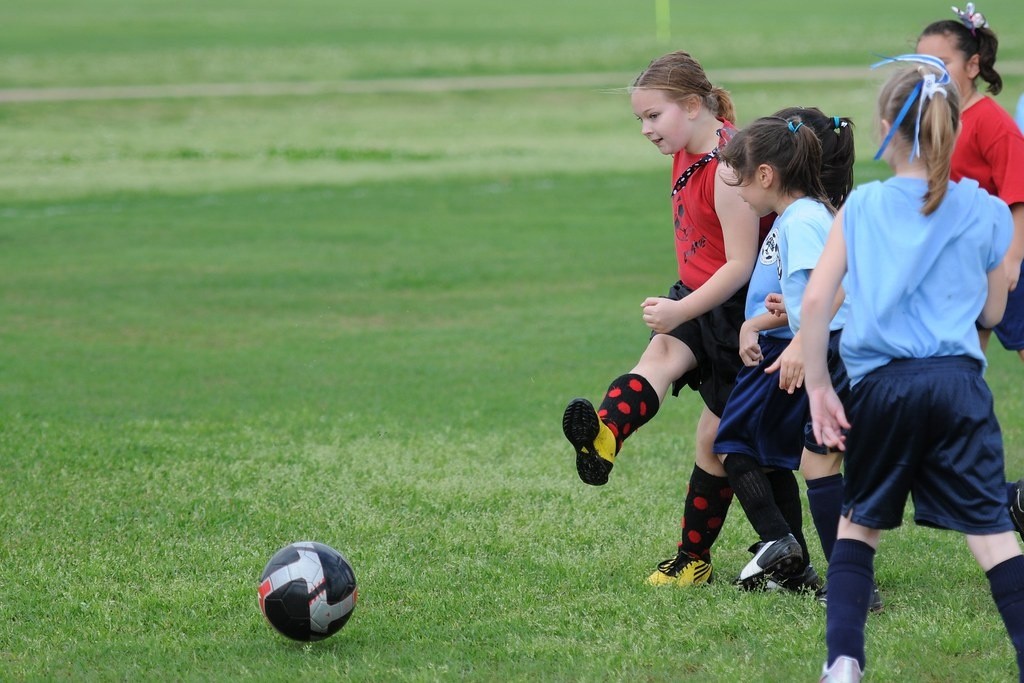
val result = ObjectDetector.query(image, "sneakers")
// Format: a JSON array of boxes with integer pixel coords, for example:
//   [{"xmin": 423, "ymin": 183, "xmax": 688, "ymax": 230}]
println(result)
[
  {"xmin": 730, "ymin": 534, "xmax": 803, "ymax": 592},
  {"xmin": 642, "ymin": 551, "xmax": 712, "ymax": 588},
  {"xmin": 816, "ymin": 655, "xmax": 865, "ymax": 683},
  {"xmin": 562, "ymin": 398, "xmax": 616, "ymax": 485},
  {"xmin": 814, "ymin": 580, "xmax": 882, "ymax": 616},
  {"xmin": 779, "ymin": 563, "xmax": 819, "ymax": 593}
]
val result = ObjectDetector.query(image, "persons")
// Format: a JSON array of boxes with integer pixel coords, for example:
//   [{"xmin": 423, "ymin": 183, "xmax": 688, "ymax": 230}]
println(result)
[
  {"xmin": 715, "ymin": 105, "xmax": 855, "ymax": 593},
  {"xmin": 563, "ymin": 50, "xmax": 777, "ymax": 586},
  {"xmin": 718, "ymin": 116, "xmax": 881, "ymax": 611},
  {"xmin": 798, "ymin": 53, "xmax": 1024, "ymax": 682},
  {"xmin": 916, "ymin": 4, "xmax": 1024, "ymax": 370}
]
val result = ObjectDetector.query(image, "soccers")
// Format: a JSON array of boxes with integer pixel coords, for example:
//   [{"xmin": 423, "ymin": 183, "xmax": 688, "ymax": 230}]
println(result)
[{"xmin": 257, "ymin": 540, "xmax": 360, "ymax": 642}]
